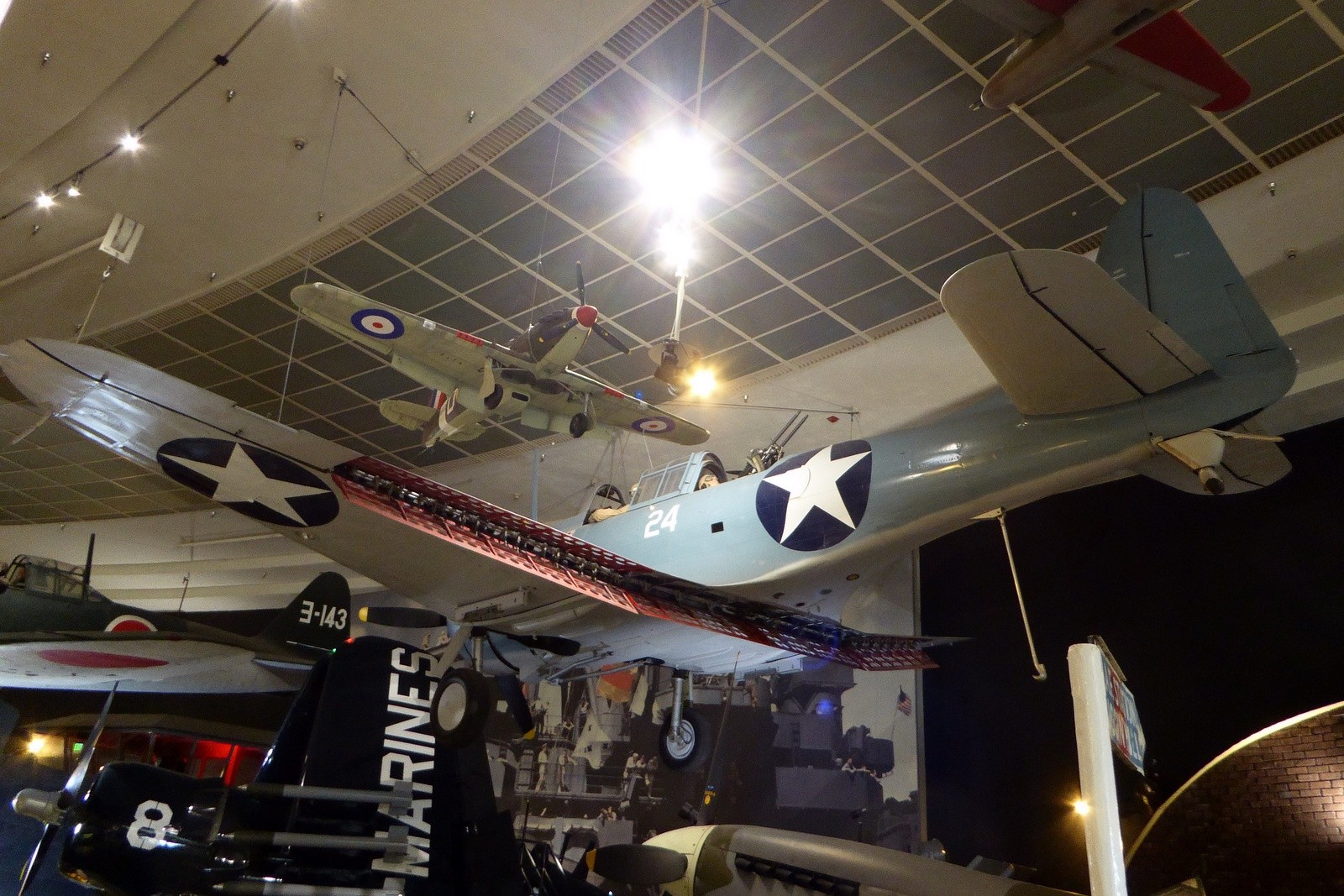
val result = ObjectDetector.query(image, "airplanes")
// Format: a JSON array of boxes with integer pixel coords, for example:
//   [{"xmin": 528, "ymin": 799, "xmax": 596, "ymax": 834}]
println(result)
[
  {"xmin": 11, "ymin": 567, "xmax": 566, "ymax": 895},
  {"xmin": 0, "ymin": 187, "xmax": 1300, "ymax": 771},
  {"xmin": 290, "ymin": 260, "xmax": 713, "ymax": 455}
]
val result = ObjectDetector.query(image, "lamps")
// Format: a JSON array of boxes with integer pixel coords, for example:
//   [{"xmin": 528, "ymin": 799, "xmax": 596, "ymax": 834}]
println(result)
[
  {"xmin": 122, "ymin": 126, "xmax": 147, "ymax": 152},
  {"xmin": 67, "ymin": 172, "xmax": 83, "ymax": 196},
  {"xmin": 41, "ymin": 184, "xmax": 59, "ymax": 206},
  {"xmin": 650, "ymin": 276, "xmax": 724, "ymax": 407}
]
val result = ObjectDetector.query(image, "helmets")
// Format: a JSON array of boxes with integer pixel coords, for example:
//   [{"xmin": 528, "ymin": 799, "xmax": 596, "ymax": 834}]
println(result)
[
  {"xmin": 629, "ymin": 483, "xmax": 639, "ymax": 498},
  {"xmin": 698, "ymin": 475, "xmax": 720, "ymax": 490}
]
